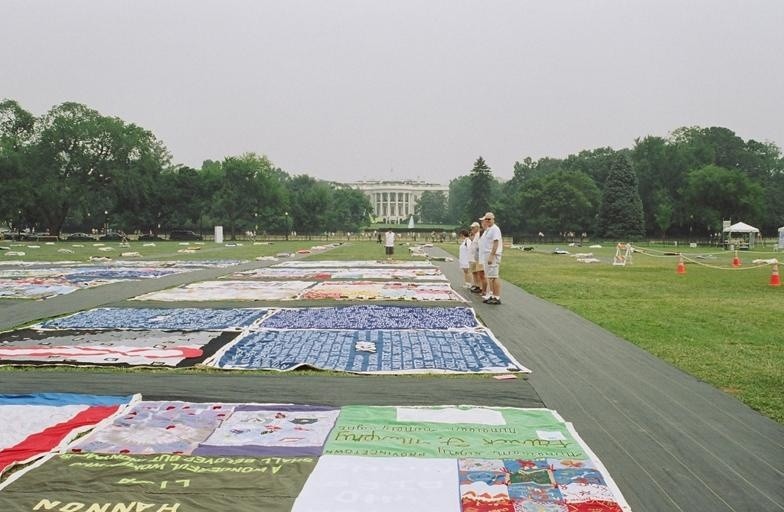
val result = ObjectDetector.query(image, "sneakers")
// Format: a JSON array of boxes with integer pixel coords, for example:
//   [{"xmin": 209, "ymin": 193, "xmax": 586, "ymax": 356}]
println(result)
[{"xmin": 462, "ymin": 282, "xmax": 501, "ymax": 304}]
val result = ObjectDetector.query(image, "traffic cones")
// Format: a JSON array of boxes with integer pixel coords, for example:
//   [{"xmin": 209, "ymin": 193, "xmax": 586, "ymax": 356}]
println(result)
[
  {"xmin": 732, "ymin": 249, "xmax": 741, "ymax": 266},
  {"xmin": 674, "ymin": 254, "xmax": 687, "ymax": 274},
  {"xmin": 767, "ymin": 262, "xmax": 783, "ymax": 287}
]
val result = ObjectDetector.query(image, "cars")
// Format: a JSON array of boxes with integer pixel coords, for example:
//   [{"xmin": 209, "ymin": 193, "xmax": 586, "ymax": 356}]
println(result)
[{"xmin": 0, "ymin": 230, "xmax": 201, "ymax": 243}]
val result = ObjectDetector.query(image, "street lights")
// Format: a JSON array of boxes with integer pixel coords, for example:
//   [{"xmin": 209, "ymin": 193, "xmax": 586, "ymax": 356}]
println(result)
[
  {"xmin": 284, "ymin": 212, "xmax": 289, "ymax": 240},
  {"xmin": 87, "ymin": 213, "xmax": 90, "ymax": 237},
  {"xmin": 104, "ymin": 211, "xmax": 108, "ymax": 237},
  {"xmin": 18, "ymin": 209, "xmax": 23, "ymax": 242},
  {"xmin": 254, "ymin": 213, "xmax": 257, "ymax": 235}
]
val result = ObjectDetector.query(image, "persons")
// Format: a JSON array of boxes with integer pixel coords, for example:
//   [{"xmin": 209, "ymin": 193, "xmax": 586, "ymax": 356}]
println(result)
[
  {"xmin": 480, "ymin": 213, "xmax": 502, "ymax": 305},
  {"xmin": 382, "ymin": 228, "xmax": 395, "ymax": 260},
  {"xmin": 344, "ymin": 229, "xmax": 462, "ymax": 245},
  {"xmin": 457, "ymin": 229, "xmax": 472, "ymax": 290},
  {"xmin": 466, "ymin": 216, "xmax": 494, "ymax": 300}
]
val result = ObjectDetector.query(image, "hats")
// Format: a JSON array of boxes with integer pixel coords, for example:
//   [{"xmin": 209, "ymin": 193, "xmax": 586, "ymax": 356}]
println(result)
[
  {"xmin": 470, "ymin": 222, "xmax": 480, "ymax": 227},
  {"xmin": 478, "ymin": 216, "xmax": 486, "ymax": 220}
]
